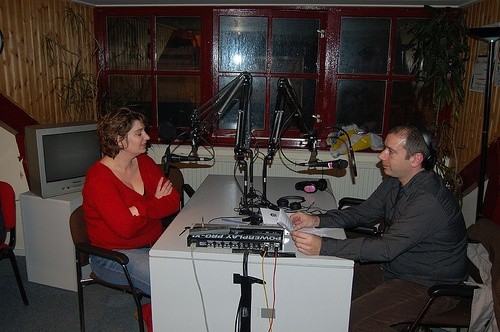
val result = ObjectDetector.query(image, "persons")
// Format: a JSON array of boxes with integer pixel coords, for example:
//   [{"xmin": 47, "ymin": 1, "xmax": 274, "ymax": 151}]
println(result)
[
  {"xmin": 82, "ymin": 107, "xmax": 180, "ymax": 332},
  {"xmin": 289, "ymin": 124, "xmax": 468, "ymax": 332},
  {"xmin": 454, "ymin": 137, "xmax": 500, "ymax": 332}
]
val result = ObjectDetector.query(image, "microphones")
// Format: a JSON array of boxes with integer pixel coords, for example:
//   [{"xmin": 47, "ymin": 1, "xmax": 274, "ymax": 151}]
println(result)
[
  {"xmin": 164, "ymin": 154, "xmax": 211, "ymax": 160},
  {"xmin": 350, "ymin": 145, "xmax": 357, "ymax": 176},
  {"xmin": 300, "ymin": 160, "xmax": 348, "ymax": 169}
]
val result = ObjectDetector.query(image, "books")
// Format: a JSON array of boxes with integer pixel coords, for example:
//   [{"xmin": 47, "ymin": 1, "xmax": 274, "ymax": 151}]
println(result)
[{"xmin": 259, "ymin": 207, "xmax": 297, "ymax": 236}]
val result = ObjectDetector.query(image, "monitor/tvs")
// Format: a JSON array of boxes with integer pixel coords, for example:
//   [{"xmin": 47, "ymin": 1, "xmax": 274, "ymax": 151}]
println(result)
[{"xmin": 24, "ymin": 121, "xmax": 102, "ymax": 199}]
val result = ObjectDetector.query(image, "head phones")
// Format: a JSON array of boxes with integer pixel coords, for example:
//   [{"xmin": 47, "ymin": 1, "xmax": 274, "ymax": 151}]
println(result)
[
  {"xmin": 295, "ymin": 179, "xmax": 327, "ymax": 193},
  {"xmin": 276, "ymin": 194, "xmax": 307, "ymax": 210}
]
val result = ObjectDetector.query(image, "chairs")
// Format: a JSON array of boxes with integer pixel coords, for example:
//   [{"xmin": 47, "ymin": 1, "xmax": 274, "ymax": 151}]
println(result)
[
  {"xmin": 69, "ymin": 206, "xmax": 146, "ymax": 332},
  {"xmin": 157, "ymin": 163, "xmax": 195, "ymax": 230},
  {"xmin": 0, "ymin": 181, "xmax": 30, "ymax": 307},
  {"xmin": 338, "ymin": 197, "xmax": 500, "ymax": 332}
]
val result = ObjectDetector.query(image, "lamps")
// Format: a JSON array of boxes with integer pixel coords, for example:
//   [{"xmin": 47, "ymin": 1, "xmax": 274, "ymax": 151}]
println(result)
[{"xmin": 468, "ymin": 27, "xmax": 500, "ymax": 222}]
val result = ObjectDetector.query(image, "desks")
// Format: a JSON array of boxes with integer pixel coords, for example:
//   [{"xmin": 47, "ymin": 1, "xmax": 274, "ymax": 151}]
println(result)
[
  {"xmin": 19, "ymin": 191, "xmax": 91, "ymax": 293},
  {"xmin": 148, "ymin": 173, "xmax": 355, "ymax": 332}
]
